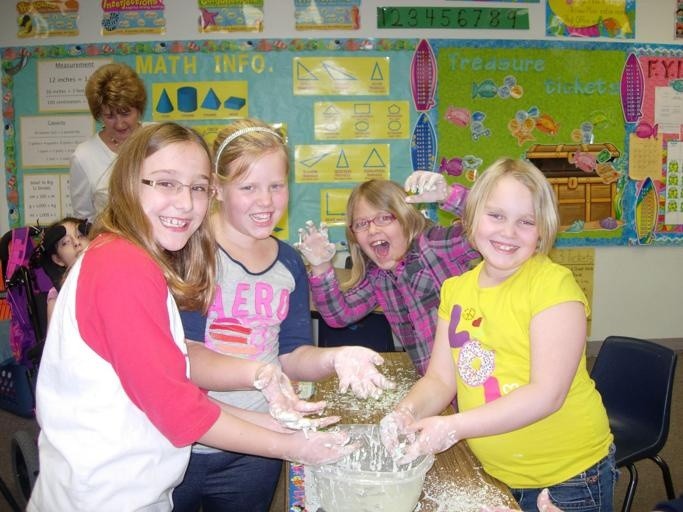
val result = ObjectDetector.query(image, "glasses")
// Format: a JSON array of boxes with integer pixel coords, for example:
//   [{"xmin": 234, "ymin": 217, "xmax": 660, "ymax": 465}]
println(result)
[
  {"xmin": 349, "ymin": 213, "xmax": 396, "ymax": 233},
  {"xmin": 140, "ymin": 179, "xmax": 217, "ymax": 200}
]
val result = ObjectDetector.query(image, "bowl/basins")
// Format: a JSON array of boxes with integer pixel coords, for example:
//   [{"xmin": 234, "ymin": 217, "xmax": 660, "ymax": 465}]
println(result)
[{"xmin": 302, "ymin": 423, "xmax": 436, "ymax": 512}]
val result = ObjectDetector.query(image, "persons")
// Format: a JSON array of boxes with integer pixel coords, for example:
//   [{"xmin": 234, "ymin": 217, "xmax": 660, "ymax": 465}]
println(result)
[
  {"xmin": 47, "ymin": 217, "xmax": 93, "ymax": 324},
  {"xmin": 376, "ymin": 157, "xmax": 618, "ymax": 508},
  {"xmin": 67, "ymin": 61, "xmax": 161, "ymax": 224},
  {"xmin": 25, "ymin": 122, "xmax": 364, "ymax": 507},
  {"xmin": 294, "ymin": 170, "xmax": 484, "ymax": 404},
  {"xmin": 174, "ymin": 124, "xmax": 396, "ymax": 507}
]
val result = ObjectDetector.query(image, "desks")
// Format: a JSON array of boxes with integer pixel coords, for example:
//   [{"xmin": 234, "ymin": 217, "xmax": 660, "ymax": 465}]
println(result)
[{"xmin": 268, "ymin": 348, "xmax": 524, "ymax": 511}]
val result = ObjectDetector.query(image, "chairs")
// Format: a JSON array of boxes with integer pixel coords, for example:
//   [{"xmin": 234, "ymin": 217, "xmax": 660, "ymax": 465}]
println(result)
[{"xmin": 586, "ymin": 335, "xmax": 679, "ymax": 512}]
